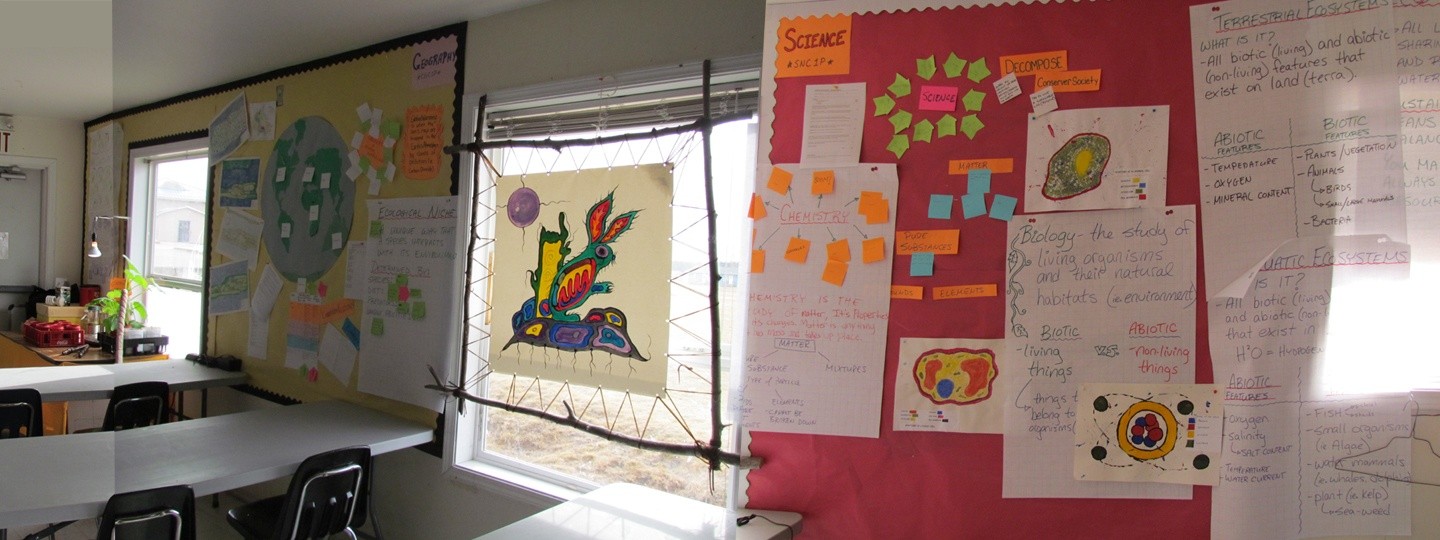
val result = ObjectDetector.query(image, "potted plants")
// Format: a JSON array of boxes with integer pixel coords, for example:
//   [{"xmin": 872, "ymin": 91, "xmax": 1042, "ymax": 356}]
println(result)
[{"xmin": 83, "ymin": 253, "xmax": 147, "ymax": 337}]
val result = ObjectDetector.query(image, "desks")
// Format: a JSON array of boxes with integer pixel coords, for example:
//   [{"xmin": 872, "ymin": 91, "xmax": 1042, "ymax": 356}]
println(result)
[
  {"xmin": 0, "ymin": 358, "xmax": 255, "ymax": 422},
  {"xmin": 472, "ymin": 480, "xmax": 803, "ymax": 540},
  {"xmin": 1, "ymin": 397, "xmax": 435, "ymax": 540},
  {"xmin": 0, "ymin": 330, "xmax": 169, "ymax": 369}
]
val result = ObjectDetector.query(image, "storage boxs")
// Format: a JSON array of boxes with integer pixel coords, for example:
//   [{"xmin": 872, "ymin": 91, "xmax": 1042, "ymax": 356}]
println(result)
[
  {"xmin": 36, "ymin": 302, "xmax": 88, "ymax": 334},
  {"xmin": 97, "ymin": 332, "xmax": 169, "ymax": 357},
  {"xmin": 23, "ymin": 323, "xmax": 85, "ymax": 348}
]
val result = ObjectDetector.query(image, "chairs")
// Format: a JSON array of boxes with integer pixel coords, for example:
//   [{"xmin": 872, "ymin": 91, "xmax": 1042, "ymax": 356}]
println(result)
[
  {"xmin": 74, "ymin": 380, "xmax": 172, "ymax": 434},
  {"xmin": 94, "ymin": 484, "xmax": 197, "ymax": 540},
  {"xmin": 225, "ymin": 444, "xmax": 383, "ymax": 540},
  {"xmin": 0, "ymin": 388, "xmax": 43, "ymax": 439}
]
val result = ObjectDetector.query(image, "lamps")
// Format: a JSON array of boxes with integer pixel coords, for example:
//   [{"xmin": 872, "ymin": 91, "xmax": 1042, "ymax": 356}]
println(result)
[{"xmin": 86, "ymin": 214, "xmax": 129, "ymax": 258}]
